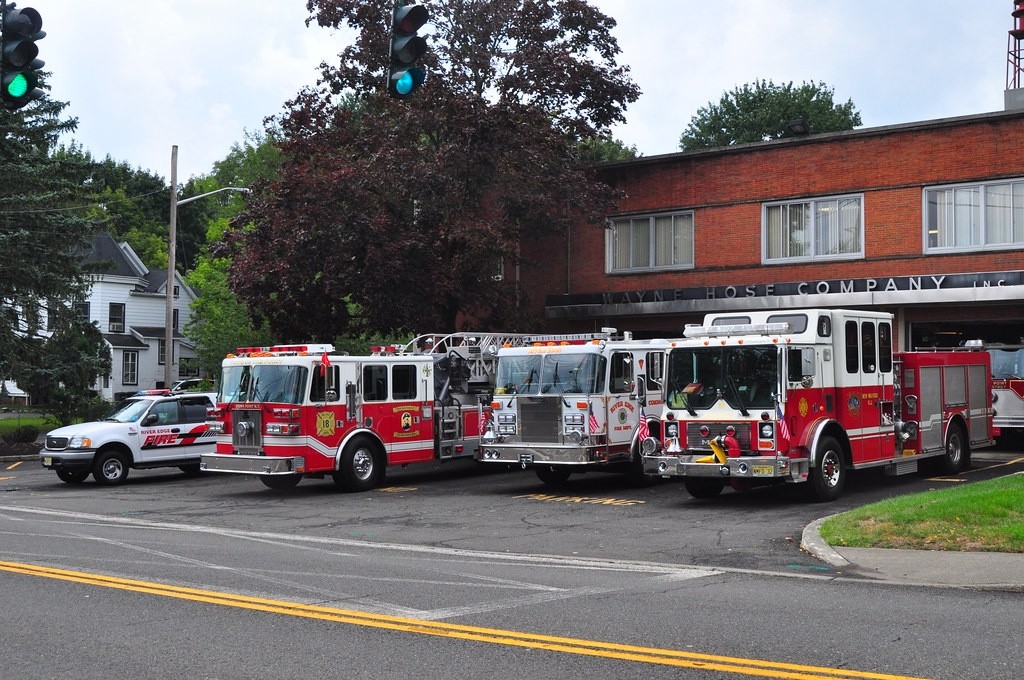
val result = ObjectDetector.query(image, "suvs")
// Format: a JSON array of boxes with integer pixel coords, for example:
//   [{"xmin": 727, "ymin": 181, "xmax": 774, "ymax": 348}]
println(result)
[
  {"xmin": 171, "ymin": 380, "xmax": 220, "ymax": 392},
  {"xmin": 38, "ymin": 386, "xmax": 219, "ymax": 486}
]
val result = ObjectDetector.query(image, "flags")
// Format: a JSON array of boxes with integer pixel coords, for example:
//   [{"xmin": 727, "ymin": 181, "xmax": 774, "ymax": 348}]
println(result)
[
  {"xmin": 776, "ymin": 404, "xmax": 790, "ymax": 441},
  {"xmin": 478, "ymin": 403, "xmax": 485, "ymax": 433},
  {"xmin": 638, "ymin": 405, "xmax": 650, "ymax": 442},
  {"xmin": 320, "ymin": 351, "xmax": 332, "ymax": 376},
  {"xmin": 589, "ymin": 404, "xmax": 600, "ymax": 434}
]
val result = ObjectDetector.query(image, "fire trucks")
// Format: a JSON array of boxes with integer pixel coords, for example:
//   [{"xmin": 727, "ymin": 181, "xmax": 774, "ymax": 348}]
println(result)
[
  {"xmin": 199, "ymin": 328, "xmax": 634, "ymax": 491},
  {"xmin": 473, "ymin": 333, "xmax": 665, "ymax": 486},
  {"xmin": 642, "ymin": 309, "xmax": 998, "ymax": 501},
  {"xmin": 960, "ymin": 335, "xmax": 1024, "ymax": 442}
]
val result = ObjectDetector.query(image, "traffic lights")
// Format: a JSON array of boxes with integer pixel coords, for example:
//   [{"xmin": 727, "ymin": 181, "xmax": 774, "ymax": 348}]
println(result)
[
  {"xmin": 387, "ymin": 2, "xmax": 429, "ymax": 100},
  {"xmin": 0, "ymin": 6, "xmax": 47, "ymax": 109}
]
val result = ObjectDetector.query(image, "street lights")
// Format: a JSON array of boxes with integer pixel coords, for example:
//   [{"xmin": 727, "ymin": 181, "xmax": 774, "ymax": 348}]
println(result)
[{"xmin": 165, "ymin": 187, "xmax": 253, "ymax": 387}]
